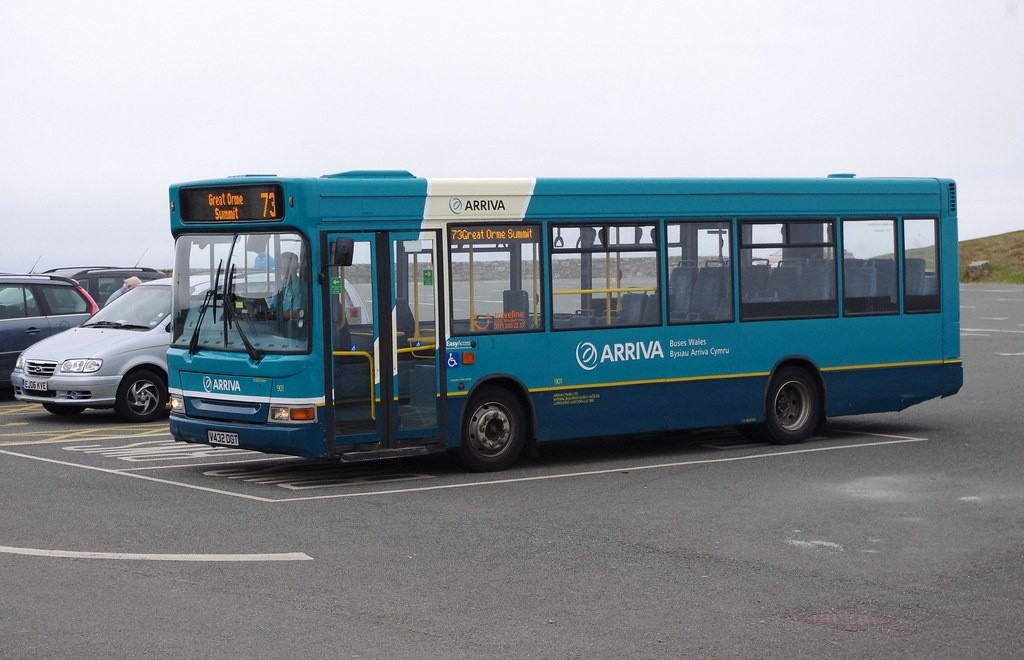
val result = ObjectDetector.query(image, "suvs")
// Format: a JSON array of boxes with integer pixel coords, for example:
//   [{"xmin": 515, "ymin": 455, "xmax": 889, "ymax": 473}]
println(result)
[
  {"xmin": 36, "ymin": 248, "xmax": 168, "ymax": 310},
  {"xmin": 0, "ymin": 255, "xmax": 102, "ymax": 399}
]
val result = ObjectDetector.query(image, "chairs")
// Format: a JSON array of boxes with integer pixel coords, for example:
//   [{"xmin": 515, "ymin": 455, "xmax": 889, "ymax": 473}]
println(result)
[
  {"xmin": 503, "ymin": 255, "xmax": 927, "ymax": 329},
  {"xmin": 0, "ymin": 304, "xmax": 35, "ymax": 319},
  {"xmin": 396, "ymin": 298, "xmax": 415, "ymax": 347}
]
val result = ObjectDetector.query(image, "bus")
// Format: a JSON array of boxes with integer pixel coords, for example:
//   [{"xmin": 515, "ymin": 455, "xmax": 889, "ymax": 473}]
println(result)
[{"xmin": 167, "ymin": 170, "xmax": 964, "ymax": 472}]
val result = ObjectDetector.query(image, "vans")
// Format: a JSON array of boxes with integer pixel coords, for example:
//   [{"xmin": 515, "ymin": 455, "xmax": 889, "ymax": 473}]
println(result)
[{"xmin": 11, "ymin": 270, "xmax": 369, "ymax": 426}]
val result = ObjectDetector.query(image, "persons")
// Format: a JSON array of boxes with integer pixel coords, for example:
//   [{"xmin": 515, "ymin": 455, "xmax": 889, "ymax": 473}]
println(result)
[
  {"xmin": 262, "ymin": 252, "xmax": 309, "ymax": 344},
  {"xmin": 104, "ymin": 276, "xmax": 144, "ymax": 311}
]
